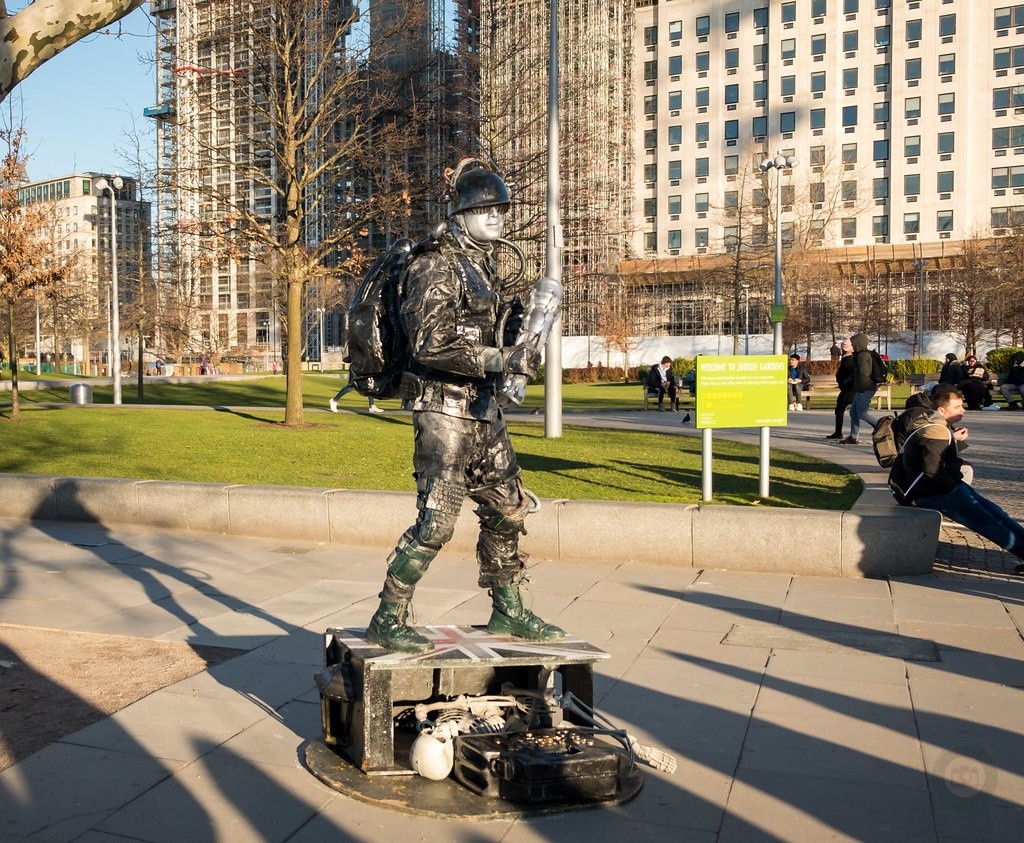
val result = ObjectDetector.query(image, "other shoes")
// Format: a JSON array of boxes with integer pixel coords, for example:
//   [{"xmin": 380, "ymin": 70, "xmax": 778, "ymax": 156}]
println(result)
[
  {"xmin": 670, "ymin": 406, "xmax": 677, "ymax": 412},
  {"xmin": 368, "ymin": 405, "xmax": 384, "ymax": 413},
  {"xmin": 329, "ymin": 398, "xmax": 338, "ymax": 413},
  {"xmin": 826, "ymin": 432, "xmax": 843, "ymax": 439},
  {"xmin": 789, "ymin": 404, "xmax": 794, "ymax": 411},
  {"xmin": 999, "ymin": 405, "xmax": 1019, "ymax": 410},
  {"xmin": 658, "ymin": 405, "xmax": 665, "ymax": 411},
  {"xmin": 968, "ymin": 404, "xmax": 981, "ymax": 410},
  {"xmin": 839, "ymin": 438, "xmax": 858, "ymax": 444},
  {"xmin": 982, "ymin": 405, "xmax": 1000, "ymax": 410},
  {"xmin": 796, "ymin": 404, "xmax": 802, "ymax": 410}
]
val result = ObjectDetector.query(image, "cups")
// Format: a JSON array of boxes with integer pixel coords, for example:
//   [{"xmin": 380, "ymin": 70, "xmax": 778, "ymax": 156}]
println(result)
[{"xmin": 678, "ymin": 380, "xmax": 682, "ymax": 387}]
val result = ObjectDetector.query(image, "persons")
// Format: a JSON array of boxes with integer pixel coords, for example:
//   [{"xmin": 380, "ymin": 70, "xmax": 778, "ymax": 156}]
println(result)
[
  {"xmin": 933, "ymin": 353, "xmax": 964, "ymax": 390},
  {"xmin": 156, "ymin": 358, "xmax": 162, "ymax": 375},
  {"xmin": 211, "ymin": 351, "xmax": 222, "ymax": 375},
  {"xmin": 826, "ymin": 332, "xmax": 878, "ymax": 444},
  {"xmin": 648, "ymin": 356, "xmax": 682, "ymax": 413},
  {"xmin": 991, "ymin": 351, "xmax": 1024, "ymax": 410},
  {"xmin": 788, "ymin": 354, "xmax": 810, "ymax": 411},
  {"xmin": 364, "ymin": 157, "xmax": 567, "ymax": 654},
  {"xmin": 888, "ymin": 388, "xmax": 1024, "ymax": 561},
  {"xmin": 273, "ymin": 361, "xmax": 277, "ymax": 375},
  {"xmin": 329, "ymin": 382, "xmax": 384, "ymax": 413},
  {"xmin": 961, "ymin": 356, "xmax": 993, "ymax": 410},
  {"xmin": 905, "ymin": 383, "xmax": 974, "ymax": 486},
  {"xmin": 199, "ymin": 350, "xmax": 209, "ymax": 375},
  {"xmin": 686, "ymin": 354, "xmax": 703, "ymax": 396}
]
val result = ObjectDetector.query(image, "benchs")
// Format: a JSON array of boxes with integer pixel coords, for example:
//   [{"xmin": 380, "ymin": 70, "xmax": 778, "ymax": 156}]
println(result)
[
  {"xmin": 910, "ymin": 374, "xmax": 1020, "ymax": 405},
  {"xmin": 642, "ymin": 375, "xmax": 892, "ymax": 411}
]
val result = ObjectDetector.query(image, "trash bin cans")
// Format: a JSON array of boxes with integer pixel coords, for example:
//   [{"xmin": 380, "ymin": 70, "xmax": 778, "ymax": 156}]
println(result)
[
  {"xmin": 312, "ymin": 364, "xmax": 319, "ymax": 370},
  {"xmin": 70, "ymin": 383, "xmax": 93, "ymax": 404}
]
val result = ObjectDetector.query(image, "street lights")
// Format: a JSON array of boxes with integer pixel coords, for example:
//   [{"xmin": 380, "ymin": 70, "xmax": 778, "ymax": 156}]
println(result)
[
  {"xmin": 759, "ymin": 148, "xmax": 800, "ymax": 355},
  {"xmin": 94, "ymin": 177, "xmax": 125, "ymax": 405}
]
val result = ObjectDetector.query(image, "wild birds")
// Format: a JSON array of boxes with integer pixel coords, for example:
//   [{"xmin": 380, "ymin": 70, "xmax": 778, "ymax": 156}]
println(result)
[
  {"xmin": 530, "ymin": 406, "xmax": 539, "ymax": 415},
  {"xmin": 682, "ymin": 413, "xmax": 691, "ymax": 423}
]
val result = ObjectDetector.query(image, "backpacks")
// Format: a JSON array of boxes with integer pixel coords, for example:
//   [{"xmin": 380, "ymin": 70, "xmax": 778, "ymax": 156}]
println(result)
[
  {"xmin": 872, "ymin": 407, "xmax": 952, "ymax": 469},
  {"xmin": 855, "ymin": 349, "xmax": 887, "ymax": 383}
]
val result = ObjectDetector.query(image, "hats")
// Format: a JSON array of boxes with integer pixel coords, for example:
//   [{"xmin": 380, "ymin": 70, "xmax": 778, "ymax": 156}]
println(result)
[{"xmin": 841, "ymin": 339, "xmax": 853, "ymax": 352}]
